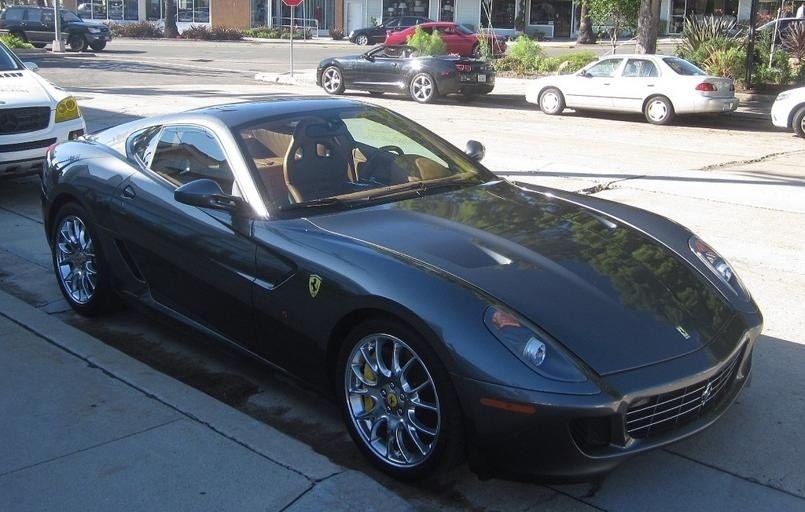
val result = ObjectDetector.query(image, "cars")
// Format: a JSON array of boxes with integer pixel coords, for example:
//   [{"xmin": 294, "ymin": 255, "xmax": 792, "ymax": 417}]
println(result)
[
  {"xmin": 348, "ymin": 16, "xmax": 508, "ymax": 56},
  {"xmin": 0, "ymin": 39, "xmax": 88, "ymax": 189},
  {"xmin": 525, "ymin": 54, "xmax": 739, "ymax": 125},
  {"xmin": 771, "ymin": 87, "xmax": 805, "ymax": 138},
  {"xmin": 0, "ymin": 5, "xmax": 113, "ymax": 52}
]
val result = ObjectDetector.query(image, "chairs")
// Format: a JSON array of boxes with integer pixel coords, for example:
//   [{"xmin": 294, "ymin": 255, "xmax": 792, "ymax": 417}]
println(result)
[
  {"xmin": 232, "ymin": 167, "xmax": 281, "ymax": 211},
  {"xmin": 284, "ymin": 116, "xmax": 389, "ymax": 205}
]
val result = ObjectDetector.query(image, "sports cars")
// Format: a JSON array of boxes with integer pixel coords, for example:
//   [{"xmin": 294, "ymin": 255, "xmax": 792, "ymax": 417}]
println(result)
[
  {"xmin": 315, "ymin": 44, "xmax": 496, "ymax": 104},
  {"xmin": 41, "ymin": 98, "xmax": 763, "ymax": 483}
]
